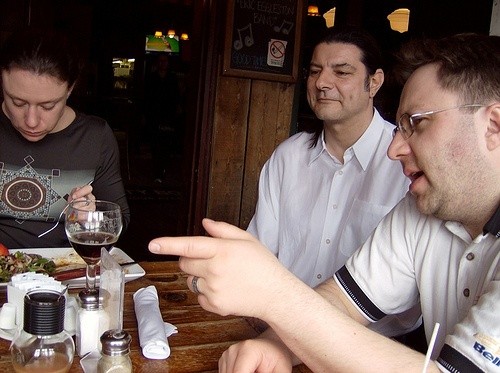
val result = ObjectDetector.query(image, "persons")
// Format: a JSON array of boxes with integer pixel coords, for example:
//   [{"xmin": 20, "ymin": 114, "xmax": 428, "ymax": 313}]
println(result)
[
  {"xmin": 0, "ymin": 21, "xmax": 129, "ymax": 249},
  {"xmin": 247, "ymin": 19, "xmax": 412, "ymax": 293},
  {"xmin": 149, "ymin": 35, "xmax": 500, "ymax": 373}
]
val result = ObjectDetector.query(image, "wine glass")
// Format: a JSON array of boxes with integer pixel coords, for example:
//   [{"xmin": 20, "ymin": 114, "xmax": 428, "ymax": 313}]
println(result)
[{"xmin": 64, "ymin": 200, "xmax": 123, "ymax": 311}]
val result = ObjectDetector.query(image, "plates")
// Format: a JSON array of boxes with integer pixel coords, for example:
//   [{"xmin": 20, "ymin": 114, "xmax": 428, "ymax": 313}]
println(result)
[
  {"xmin": 0, "ymin": 295, "xmax": 78, "ymax": 341},
  {"xmin": 0, "ymin": 244, "xmax": 146, "ymax": 294}
]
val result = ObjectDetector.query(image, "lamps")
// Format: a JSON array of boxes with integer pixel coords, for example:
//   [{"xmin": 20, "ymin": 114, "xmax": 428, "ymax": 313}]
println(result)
[
  {"xmin": 307, "ymin": 4, "xmax": 321, "ymax": 17},
  {"xmin": 144, "ymin": 0, "xmax": 192, "ymax": 41}
]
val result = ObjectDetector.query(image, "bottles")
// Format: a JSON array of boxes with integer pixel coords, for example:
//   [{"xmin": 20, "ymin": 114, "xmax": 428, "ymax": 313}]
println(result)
[
  {"xmin": 97, "ymin": 330, "xmax": 134, "ymax": 373},
  {"xmin": 10, "ymin": 289, "xmax": 76, "ymax": 373},
  {"xmin": 74, "ymin": 288, "xmax": 111, "ymax": 358}
]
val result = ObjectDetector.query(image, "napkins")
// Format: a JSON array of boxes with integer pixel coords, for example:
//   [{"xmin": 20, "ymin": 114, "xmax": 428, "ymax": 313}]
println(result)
[{"xmin": 133, "ymin": 285, "xmax": 178, "ymax": 360}]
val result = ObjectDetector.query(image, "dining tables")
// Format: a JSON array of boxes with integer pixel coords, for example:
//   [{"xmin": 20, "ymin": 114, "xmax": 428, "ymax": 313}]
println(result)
[{"xmin": 0, "ymin": 261, "xmax": 314, "ymax": 373}]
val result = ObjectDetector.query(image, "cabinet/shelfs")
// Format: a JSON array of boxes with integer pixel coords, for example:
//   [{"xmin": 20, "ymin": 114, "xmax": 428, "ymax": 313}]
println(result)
[{"xmin": 84, "ymin": 95, "xmax": 131, "ymax": 179}]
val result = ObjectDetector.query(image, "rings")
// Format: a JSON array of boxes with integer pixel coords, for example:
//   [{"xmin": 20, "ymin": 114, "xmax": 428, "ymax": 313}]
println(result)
[
  {"xmin": 192, "ymin": 276, "xmax": 202, "ymax": 295},
  {"xmin": 84, "ymin": 196, "xmax": 92, "ymax": 207}
]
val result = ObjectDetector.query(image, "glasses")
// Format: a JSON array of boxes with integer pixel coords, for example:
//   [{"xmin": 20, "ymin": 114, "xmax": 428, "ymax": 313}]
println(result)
[{"xmin": 392, "ymin": 103, "xmax": 491, "ymax": 139}]
